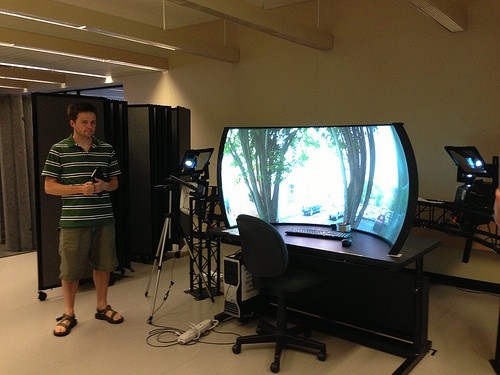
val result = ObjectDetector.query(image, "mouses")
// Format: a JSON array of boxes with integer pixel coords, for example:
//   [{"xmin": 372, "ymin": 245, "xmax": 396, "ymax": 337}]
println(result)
[{"xmin": 342, "ymin": 238, "xmax": 353, "ymax": 247}]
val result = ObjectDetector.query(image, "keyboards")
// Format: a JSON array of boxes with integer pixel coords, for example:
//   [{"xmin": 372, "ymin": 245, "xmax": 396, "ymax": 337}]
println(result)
[{"xmin": 285, "ymin": 226, "xmax": 352, "ymax": 240}]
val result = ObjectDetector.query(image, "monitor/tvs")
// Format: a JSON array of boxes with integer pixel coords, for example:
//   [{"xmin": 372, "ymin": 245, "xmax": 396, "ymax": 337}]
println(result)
[{"xmin": 217, "ymin": 122, "xmax": 419, "ymax": 255}]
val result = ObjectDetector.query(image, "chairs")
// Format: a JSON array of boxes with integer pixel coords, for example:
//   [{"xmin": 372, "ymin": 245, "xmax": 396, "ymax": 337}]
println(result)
[{"xmin": 232, "ymin": 214, "xmax": 326, "ymax": 373}]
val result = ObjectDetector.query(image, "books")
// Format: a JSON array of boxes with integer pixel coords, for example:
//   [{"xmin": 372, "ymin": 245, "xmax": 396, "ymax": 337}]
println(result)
[{"xmin": 90, "ymin": 166, "xmax": 108, "ymax": 197}]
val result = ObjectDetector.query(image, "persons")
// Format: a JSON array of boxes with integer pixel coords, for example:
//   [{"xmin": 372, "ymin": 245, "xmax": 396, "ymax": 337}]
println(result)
[{"xmin": 39, "ymin": 101, "xmax": 126, "ymax": 338}]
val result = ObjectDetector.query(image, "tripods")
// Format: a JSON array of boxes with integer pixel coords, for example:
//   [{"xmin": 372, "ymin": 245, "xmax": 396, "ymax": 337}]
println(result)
[{"xmin": 144, "ymin": 187, "xmax": 215, "ymax": 325}]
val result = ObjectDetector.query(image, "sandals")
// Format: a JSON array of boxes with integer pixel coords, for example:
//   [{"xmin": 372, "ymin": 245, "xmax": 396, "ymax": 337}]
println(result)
[
  {"xmin": 95, "ymin": 305, "xmax": 125, "ymax": 324},
  {"xmin": 53, "ymin": 314, "xmax": 78, "ymax": 337}
]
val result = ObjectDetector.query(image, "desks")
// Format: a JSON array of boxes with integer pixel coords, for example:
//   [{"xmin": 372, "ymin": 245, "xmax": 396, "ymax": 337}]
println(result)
[{"xmin": 208, "ymin": 226, "xmax": 443, "ymax": 375}]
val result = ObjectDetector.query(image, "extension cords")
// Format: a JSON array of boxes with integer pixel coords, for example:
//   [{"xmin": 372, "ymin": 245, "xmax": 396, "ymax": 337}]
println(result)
[{"xmin": 177, "ymin": 319, "xmax": 213, "ymax": 344}]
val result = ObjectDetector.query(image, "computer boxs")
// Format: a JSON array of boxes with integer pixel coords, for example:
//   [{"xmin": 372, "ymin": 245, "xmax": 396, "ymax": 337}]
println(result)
[{"xmin": 223, "ymin": 249, "xmax": 262, "ymax": 318}]
